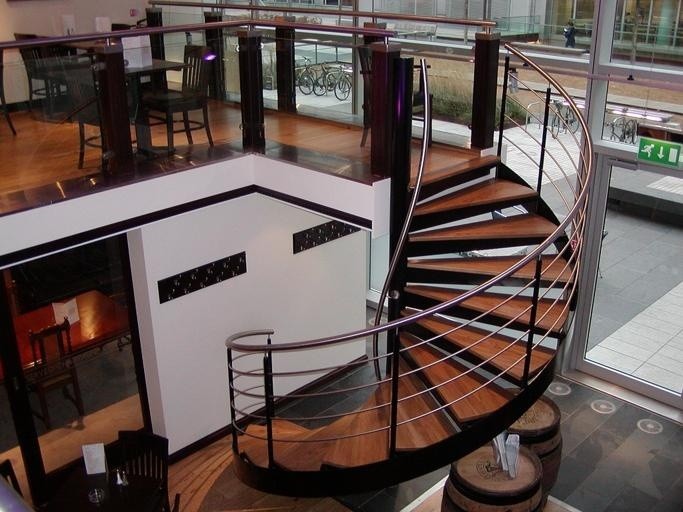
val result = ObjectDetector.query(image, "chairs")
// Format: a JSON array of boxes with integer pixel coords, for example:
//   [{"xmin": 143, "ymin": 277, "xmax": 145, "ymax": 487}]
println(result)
[
  {"xmin": 0, "ymin": 459, "xmax": 22, "ymax": 498},
  {"xmin": 21, "ymin": 318, "xmax": 85, "ymax": 431},
  {"xmin": 0, "ymin": 23, "xmax": 222, "ymax": 171},
  {"xmin": 171, "ymin": 491, "xmax": 181, "ymax": 511},
  {"xmin": 105, "ymin": 425, "xmax": 174, "ymax": 506}
]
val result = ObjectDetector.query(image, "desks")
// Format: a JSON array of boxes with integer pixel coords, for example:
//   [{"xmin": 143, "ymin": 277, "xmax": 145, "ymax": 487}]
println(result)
[{"xmin": 32, "ymin": 466, "xmax": 166, "ymax": 512}]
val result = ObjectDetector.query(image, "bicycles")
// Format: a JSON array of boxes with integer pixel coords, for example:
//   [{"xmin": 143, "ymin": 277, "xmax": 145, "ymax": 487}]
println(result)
[
  {"xmin": 298, "ymin": 55, "xmax": 314, "ymax": 96},
  {"xmin": 333, "ymin": 62, "xmax": 352, "ymax": 101},
  {"xmin": 607, "ymin": 108, "xmax": 637, "ymax": 144},
  {"xmin": 543, "ymin": 95, "xmax": 579, "ymax": 138},
  {"xmin": 312, "ymin": 60, "xmax": 335, "ymax": 96}
]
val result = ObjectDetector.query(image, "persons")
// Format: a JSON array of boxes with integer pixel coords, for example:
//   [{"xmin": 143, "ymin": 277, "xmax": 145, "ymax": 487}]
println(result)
[{"xmin": 563, "ymin": 20, "xmax": 575, "ymax": 48}]
